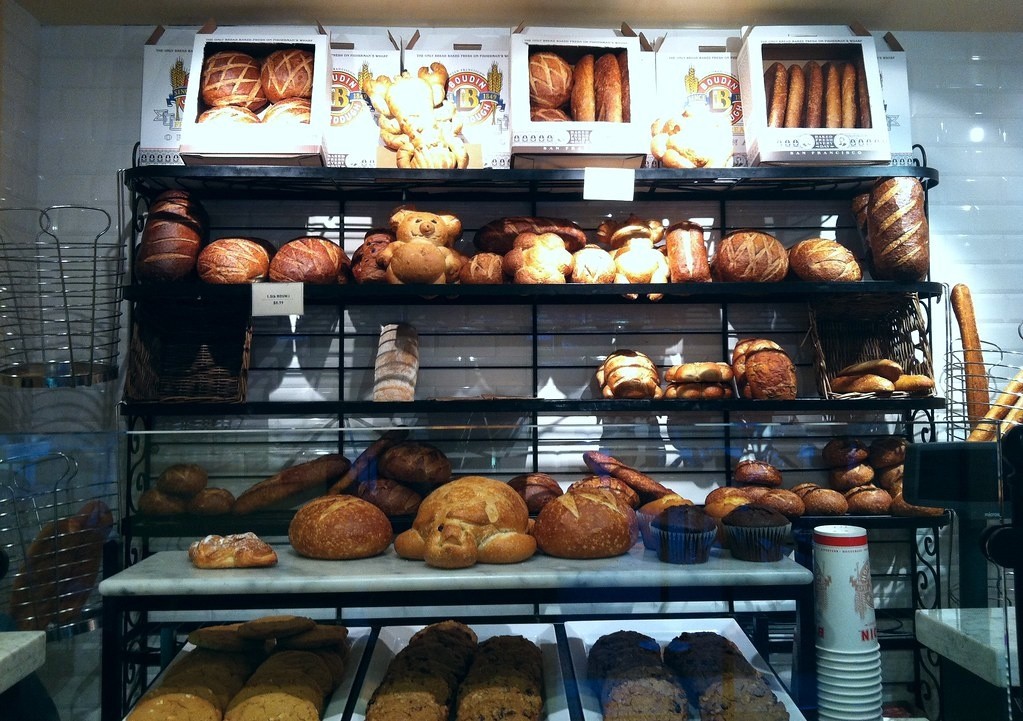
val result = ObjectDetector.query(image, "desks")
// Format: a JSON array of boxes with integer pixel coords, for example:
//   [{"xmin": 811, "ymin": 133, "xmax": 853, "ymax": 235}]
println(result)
[{"xmin": 913, "ymin": 607, "xmax": 1020, "ymax": 689}]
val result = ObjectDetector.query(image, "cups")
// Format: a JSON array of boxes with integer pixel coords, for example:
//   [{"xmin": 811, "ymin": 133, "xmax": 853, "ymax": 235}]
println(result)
[{"xmin": 813, "ymin": 525, "xmax": 884, "ymax": 721}]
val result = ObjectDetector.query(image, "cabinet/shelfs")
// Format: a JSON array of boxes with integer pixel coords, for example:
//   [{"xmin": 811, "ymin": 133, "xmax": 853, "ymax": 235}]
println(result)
[
  {"xmin": 98, "ymin": 536, "xmax": 814, "ymax": 721},
  {"xmin": 99, "ymin": 143, "xmax": 951, "ymax": 721}
]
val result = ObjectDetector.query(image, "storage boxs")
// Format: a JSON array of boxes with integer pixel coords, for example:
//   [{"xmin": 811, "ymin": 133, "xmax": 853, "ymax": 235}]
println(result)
[
  {"xmin": 138, "ymin": 25, "xmax": 194, "ymax": 166},
  {"xmin": 656, "ymin": 31, "xmax": 747, "ymax": 153},
  {"xmin": 736, "ymin": 17, "xmax": 892, "ymax": 167},
  {"xmin": 327, "ymin": 27, "xmax": 403, "ymax": 168},
  {"xmin": 404, "ymin": 28, "xmax": 512, "ymax": 170},
  {"xmin": 509, "ymin": 19, "xmax": 647, "ymax": 169},
  {"xmin": 640, "ymin": 32, "xmax": 656, "ymax": 154},
  {"xmin": 179, "ymin": 19, "xmax": 334, "ymax": 167},
  {"xmin": 877, "ymin": 30, "xmax": 913, "ymax": 165}
]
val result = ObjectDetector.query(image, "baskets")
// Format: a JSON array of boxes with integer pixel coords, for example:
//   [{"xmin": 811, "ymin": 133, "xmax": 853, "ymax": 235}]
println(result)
[
  {"xmin": 805, "ymin": 288, "xmax": 939, "ymax": 402},
  {"xmin": 121, "ymin": 302, "xmax": 253, "ymax": 406}
]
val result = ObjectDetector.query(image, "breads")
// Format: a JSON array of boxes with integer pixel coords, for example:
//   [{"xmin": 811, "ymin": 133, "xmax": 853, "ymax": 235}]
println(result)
[{"xmin": 0, "ymin": 50, "xmax": 1023, "ymax": 721}]
